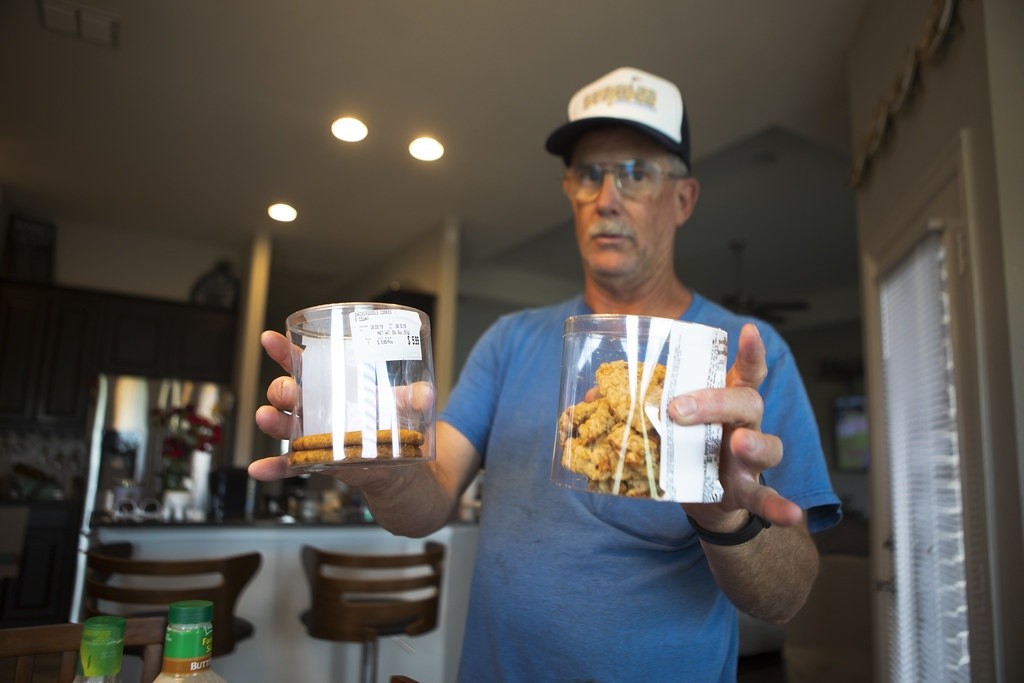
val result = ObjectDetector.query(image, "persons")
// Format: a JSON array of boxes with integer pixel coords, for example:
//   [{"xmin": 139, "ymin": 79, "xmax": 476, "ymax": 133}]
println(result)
[{"xmin": 247, "ymin": 68, "xmax": 843, "ymax": 683}]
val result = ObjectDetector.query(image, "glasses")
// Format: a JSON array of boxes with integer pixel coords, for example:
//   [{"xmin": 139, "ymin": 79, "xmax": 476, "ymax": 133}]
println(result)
[{"xmin": 567, "ymin": 161, "xmax": 689, "ymax": 202}]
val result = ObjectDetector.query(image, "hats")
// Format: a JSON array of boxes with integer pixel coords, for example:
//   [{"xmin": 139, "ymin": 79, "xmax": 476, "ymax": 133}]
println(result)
[{"xmin": 545, "ymin": 67, "xmax": 690, "ymax": 171}]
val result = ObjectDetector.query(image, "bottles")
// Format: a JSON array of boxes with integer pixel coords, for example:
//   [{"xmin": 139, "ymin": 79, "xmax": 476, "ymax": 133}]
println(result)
[
  {"xmin": 152, "ymin": 600, "xmax": 228, "ymax": 683},
  {"xmin": 72, "ymin": 616, "xmax": 128, "ymax": 683}
]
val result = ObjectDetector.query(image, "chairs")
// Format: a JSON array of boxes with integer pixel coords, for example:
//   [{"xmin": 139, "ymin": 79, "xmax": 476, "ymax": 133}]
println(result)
[
  {"xmin": 302, "ymin": 537, "xmax": 445, "ymax": 683},
  {"xmin": 1, "ymin": 622, "xmax": 86, "ymax": 682},
  {"xmin": 80, "ymin": 542, "xmax": 263, "ymax": 682}
]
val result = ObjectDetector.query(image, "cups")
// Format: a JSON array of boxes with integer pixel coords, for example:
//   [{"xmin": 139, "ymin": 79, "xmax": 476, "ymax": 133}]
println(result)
[
  {"xmin": 548, "ymin": 313, "xmax": 729, "ymax": 504},
  {"xmin": 284, "ymin": 301, "xmax": 437, "ymax": 474}
]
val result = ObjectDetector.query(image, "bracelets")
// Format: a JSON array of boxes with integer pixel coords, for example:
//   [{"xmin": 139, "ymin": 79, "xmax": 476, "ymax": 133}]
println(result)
[{"xmin": 685, "ymin": 471, "xmax": 773, "ymax": 546}]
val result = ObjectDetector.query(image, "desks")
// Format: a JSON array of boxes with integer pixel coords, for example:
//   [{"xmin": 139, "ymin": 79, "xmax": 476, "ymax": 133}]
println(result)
[{"xmin": 97, "ymin": 524, "xmax": 482, "ymax": 683}]
